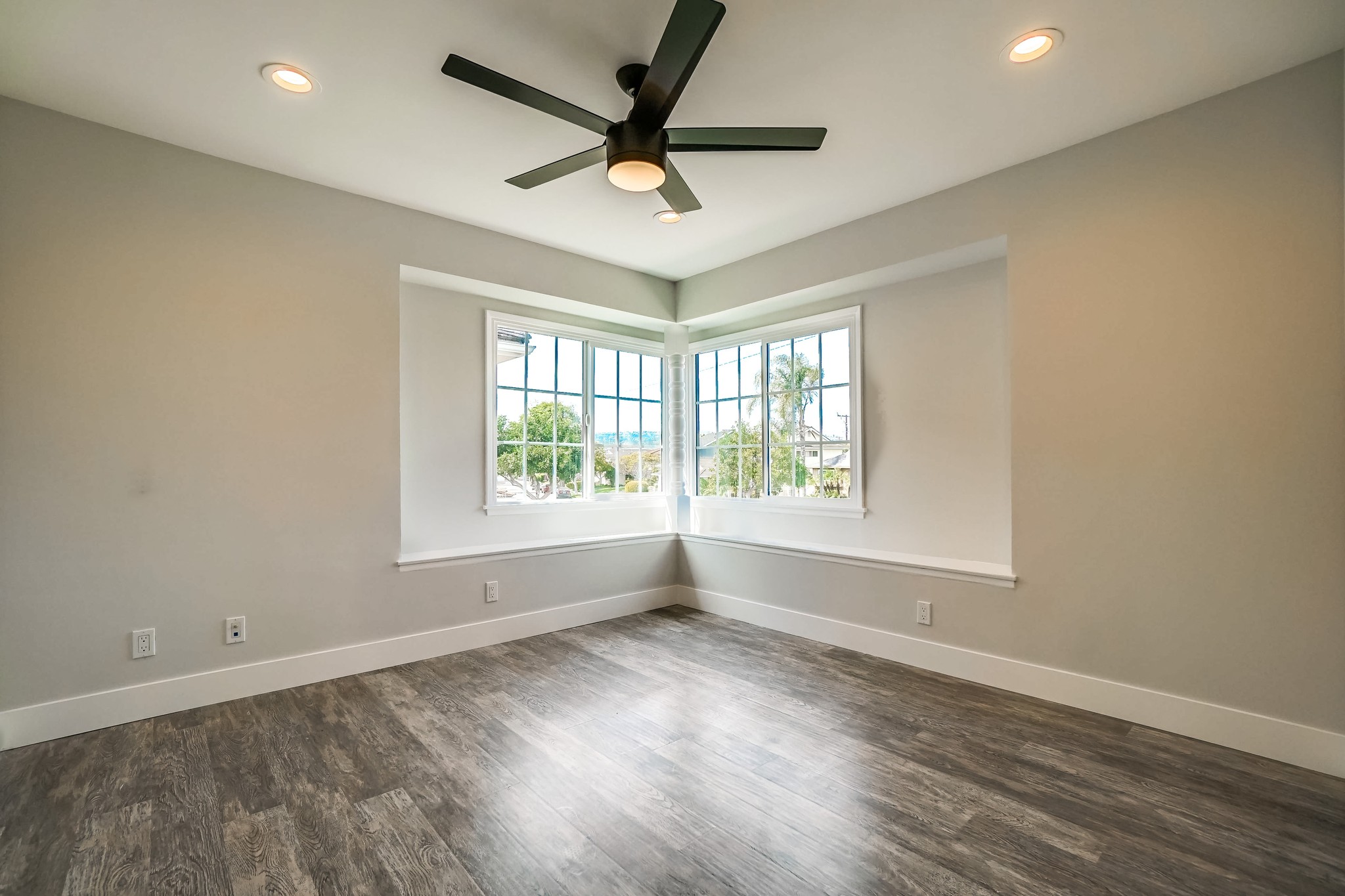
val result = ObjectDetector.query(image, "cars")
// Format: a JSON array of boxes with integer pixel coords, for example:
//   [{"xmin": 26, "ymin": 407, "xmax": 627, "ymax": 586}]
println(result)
[
  {"xmin": 527, "ymin": 477, "xmax": 531, "ymax": 481},
  {"xmin": 556, "ymin": 487, "xmax": 571, "ymax": 499},
  {"xmin": 532, "ymin": 481, "xmax": 552, "ymax": 494}
]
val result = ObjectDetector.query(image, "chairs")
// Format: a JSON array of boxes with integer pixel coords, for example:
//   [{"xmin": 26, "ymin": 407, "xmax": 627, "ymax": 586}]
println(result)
[{"xmin": 496, "ymin": 487, "xmax": 515, "ymax": 498}]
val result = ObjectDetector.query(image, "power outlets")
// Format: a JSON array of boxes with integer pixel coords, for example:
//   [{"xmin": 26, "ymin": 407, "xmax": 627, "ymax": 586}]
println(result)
[
  {"xmin": 483, "ymin": 580, "xmax": 499, "ymax": 604},
  {"xmin": 129, "ymin": 626, "xmax": 158, "ymax": 662},
  {"xmin": 916, "ymin": 599, "xmax": 932, "ymax": 627}
]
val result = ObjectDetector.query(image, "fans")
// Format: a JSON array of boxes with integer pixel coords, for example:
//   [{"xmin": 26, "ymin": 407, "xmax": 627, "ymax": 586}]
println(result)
[{"xmin": 437, "ymin": 0, "xmax": 830, "ymax": 221}]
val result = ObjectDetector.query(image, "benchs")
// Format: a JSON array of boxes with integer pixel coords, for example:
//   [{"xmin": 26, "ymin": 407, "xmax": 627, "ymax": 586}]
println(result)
[{"xmin": 557, "ymin": 486, "xmax": 571, "ymax": 498}]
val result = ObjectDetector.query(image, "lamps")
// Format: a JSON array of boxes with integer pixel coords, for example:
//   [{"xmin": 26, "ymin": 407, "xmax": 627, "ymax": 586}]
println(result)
[{"xmin": 602, "ymin": 121, "xmax": 671, "ymax": 197}]
